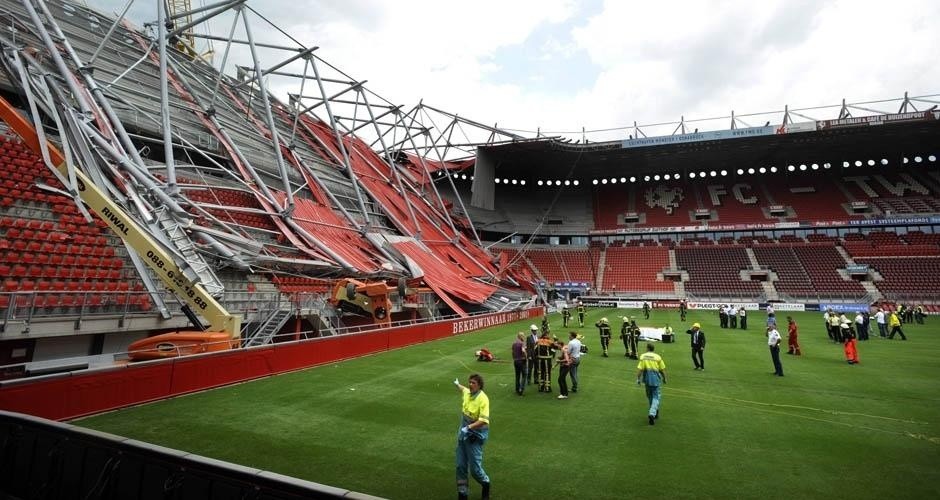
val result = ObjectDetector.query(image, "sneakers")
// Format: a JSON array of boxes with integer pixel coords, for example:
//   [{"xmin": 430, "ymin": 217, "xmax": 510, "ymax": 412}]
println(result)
[
  {"xmin": 649, "ymin": 415, "xmax": 654, "ymax": 424},
  {"xmin": 557, "ymin": 394, "xmax": 567, "ymax": 398}
]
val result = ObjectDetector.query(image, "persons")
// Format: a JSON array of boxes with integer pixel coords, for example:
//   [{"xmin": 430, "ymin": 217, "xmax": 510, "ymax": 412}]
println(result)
[
  {"xmin": 718, "ymin": 305, "xmax": 748, "ymax": 329},
  {"xmin": 763, "ymin": 301, "xmax": 801, "ymax": 376},
  {"xmin": 451, "ymin": 374, "xmax": 491, "ymax": 499},
  {"xmin": 510, "ymin": 298, "xmax": 706, "ymax": 425},
  {"xmin": 822, "ymin": 302, "xmax": 927, "ymax": 364}
]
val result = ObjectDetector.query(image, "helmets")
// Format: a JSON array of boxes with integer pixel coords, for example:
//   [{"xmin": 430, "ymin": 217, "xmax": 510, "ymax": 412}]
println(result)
[
  {"xmin": 693, "ymin": 322, "xmax": 700, "ymax": 329},
  {"xmin": 530, "ymin": 324, "xmax": 538, "ymax": 330}
]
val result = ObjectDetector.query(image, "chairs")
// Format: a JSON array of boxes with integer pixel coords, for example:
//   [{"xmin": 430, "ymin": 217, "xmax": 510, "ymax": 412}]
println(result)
[
  {"xmin": 0, "ymin": 80, "xmax": 429, "ymax": 319},
  {"xmin": 486, "ymin": 171, "xmax": 939, "ymax": 309}
]
